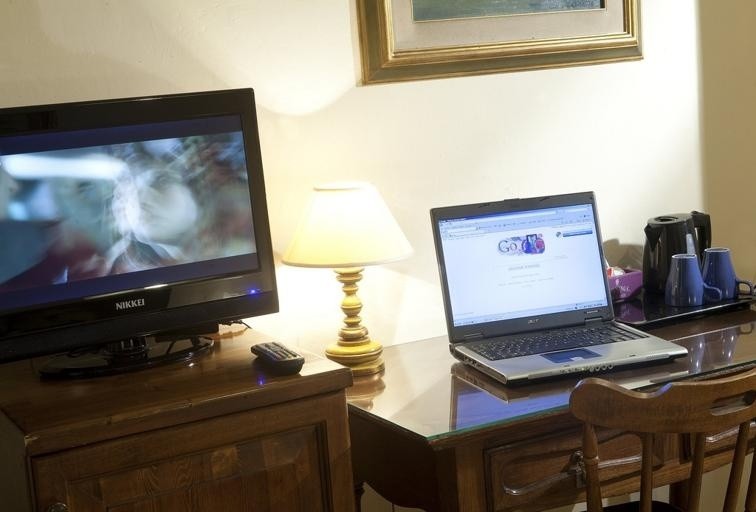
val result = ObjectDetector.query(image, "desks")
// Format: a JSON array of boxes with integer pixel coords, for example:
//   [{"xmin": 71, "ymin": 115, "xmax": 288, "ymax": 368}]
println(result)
[{"xmin": 348, "ymin": 301, "xmax": 756, "ymax": 512}]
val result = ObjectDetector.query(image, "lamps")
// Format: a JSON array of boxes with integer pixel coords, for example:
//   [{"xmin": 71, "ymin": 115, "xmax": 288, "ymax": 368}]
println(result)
[{"xmin": 278, "ymin": 177, "xmax": 415, "ymax": 377}]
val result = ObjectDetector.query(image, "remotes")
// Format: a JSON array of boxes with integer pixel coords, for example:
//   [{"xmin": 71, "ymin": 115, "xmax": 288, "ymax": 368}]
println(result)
[{"xmin": 250, "ymin": 340, "xmax": 305, "ymax": 377}]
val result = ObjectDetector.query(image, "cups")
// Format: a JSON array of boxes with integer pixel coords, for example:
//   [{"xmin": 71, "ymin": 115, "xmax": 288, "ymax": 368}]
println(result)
[{"xmin": 664, "ymin": 247, "xmax": 753, "ymax": 307}]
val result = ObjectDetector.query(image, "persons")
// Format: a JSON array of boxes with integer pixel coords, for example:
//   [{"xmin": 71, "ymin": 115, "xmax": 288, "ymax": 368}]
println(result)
[{"xmin": 96, "ymin": 139, "xmax": 256, "ymax": 271}]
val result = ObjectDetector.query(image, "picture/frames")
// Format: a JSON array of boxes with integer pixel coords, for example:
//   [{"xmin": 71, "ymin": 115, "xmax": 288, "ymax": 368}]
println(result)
[{"xmin": 350, "ymin": 0, "xmax": 644, "ymax": 87}]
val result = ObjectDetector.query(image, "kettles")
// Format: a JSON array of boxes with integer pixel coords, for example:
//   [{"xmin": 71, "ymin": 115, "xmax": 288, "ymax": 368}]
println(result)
[{"xmin": 642, "ymin": 210, "xmax": 711, "ymax": 305}]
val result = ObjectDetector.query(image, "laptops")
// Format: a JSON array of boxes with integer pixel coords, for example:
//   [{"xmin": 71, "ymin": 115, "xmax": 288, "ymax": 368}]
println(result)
[{"xmin": 430, "ymin": 190, "xmax": 689, "ymax": 387}]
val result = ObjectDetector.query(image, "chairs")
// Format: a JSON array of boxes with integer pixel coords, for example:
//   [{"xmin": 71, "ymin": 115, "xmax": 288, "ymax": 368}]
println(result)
[{"xmin": 568, "ymin": 367, "xmax": 756, "ymax": 512}]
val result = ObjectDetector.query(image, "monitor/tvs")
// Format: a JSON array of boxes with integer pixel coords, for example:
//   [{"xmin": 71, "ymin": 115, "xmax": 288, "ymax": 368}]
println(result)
[{"xmin": 0, "ymin": 87, "xmax": 280, "ymax": 382}]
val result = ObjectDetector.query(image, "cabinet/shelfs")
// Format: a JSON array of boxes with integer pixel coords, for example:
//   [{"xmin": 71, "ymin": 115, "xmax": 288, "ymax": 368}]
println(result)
[{"xmin": 2, "ymin": 319, "xmax": 357, "ymax": 512}]
